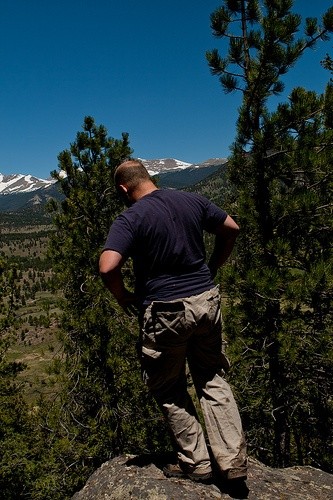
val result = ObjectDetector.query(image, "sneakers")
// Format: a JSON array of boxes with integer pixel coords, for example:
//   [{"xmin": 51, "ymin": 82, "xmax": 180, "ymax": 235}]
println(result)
[
  {"xmin": 163, "ymin": 462, "xmax": 212, "ymax": 481},
  {"xmin": 221, "ymin": 468, "xmax": 248, "ymax": 480}
]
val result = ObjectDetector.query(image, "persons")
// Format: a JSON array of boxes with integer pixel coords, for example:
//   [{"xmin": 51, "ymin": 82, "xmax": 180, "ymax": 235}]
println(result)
[{"xmin": 96, "ymin": 159, "xmax": 249, "ymax": 483}]
